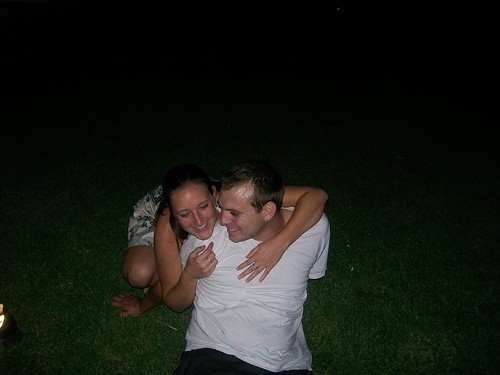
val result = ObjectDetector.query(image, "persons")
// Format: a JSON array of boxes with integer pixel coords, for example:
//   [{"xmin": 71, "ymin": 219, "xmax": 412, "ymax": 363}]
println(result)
[{"xmin": 112, "ymin": 155, "xmax": 331, "ymax": 375}]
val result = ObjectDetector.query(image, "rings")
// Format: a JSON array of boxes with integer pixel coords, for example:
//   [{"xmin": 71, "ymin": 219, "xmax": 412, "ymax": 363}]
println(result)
[
  {"xmin": 253, "ymin": 263, "xmax": 256, "ymax": 269},
  {"xmin": 206, "ymin": 258, "xmax": 212, "ymax": 263}
]
